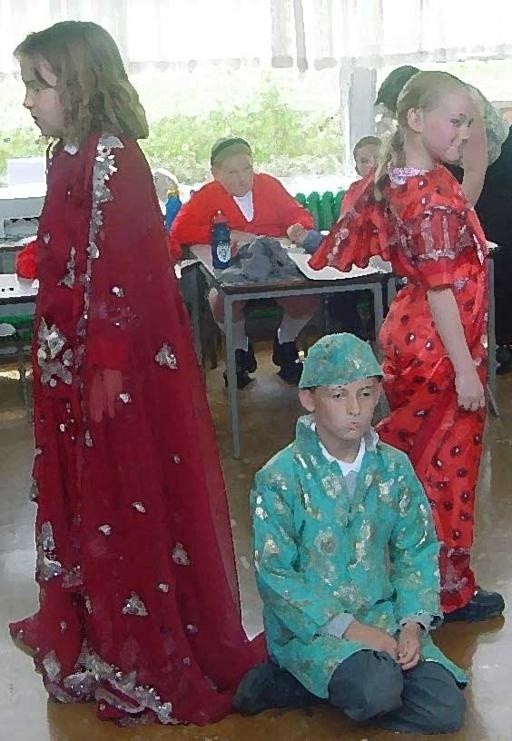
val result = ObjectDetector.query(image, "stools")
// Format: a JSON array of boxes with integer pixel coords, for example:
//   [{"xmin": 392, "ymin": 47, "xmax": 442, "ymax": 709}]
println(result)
[{"xmin": 0, "ymin": 322, "xmax": 32, "ymax": 423}]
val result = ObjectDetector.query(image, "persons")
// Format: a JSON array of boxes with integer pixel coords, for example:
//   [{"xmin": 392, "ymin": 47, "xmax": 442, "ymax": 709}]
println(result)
[
  {"xmin": 324, "ymin": 134, "xmax": 390, "ymax": 337},
  {"xmin": 173, "ymin": 135, "xmax": 316, "ymax": 383},
  {"xmin": 230, "ymin": 327, "xmax": 467, "ymax": 736},
  {"xmin": 305, "ymin": 70, "xmax": 506, "ymax": 631},
  {"xmin": 369, "ymin": 66, "xmax": 512, "ymax": 375},
  {"xmin": 13, "ymin": 17, "xmax": 267, "ymax": 729}
]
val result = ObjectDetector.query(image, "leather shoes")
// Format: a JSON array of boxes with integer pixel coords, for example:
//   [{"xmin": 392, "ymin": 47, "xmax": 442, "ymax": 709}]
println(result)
[
  {"xmin": 273, "ymin": 335, "xmax": 303, "ymax": 382},
  {"xmin": 222, "ymin": 337, "xmax": 256, "ymax": 380}
]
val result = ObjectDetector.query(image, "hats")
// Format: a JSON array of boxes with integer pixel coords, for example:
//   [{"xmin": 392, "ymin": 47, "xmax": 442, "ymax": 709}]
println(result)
[{"xmin": 299, "ymin": 333, "xmax": 384, "ymax": 390}]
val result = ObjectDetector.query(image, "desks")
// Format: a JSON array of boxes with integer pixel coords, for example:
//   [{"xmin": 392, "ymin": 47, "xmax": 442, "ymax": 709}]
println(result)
[
  {"xmin": 187, "ymin": 235, "xmax": 499, "ymax": 460},
  {"xmin": 0, "ymin": 272, "xmax": 40, "ymax": 306}
]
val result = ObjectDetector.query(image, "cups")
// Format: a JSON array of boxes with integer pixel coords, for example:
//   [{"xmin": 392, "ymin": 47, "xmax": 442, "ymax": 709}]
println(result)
[
  {"xmin": 210, "ymin": 207, "xmax": 233, "ymax": 270},
  {"xmin": 164, "ymin": 187, "xmax": 185, "ymax": 235}
]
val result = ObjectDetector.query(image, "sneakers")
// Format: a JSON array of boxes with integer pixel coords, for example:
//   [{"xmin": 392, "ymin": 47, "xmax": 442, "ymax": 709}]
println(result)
[
  {"xmin": 438, "ymin": 585, "xmax": 504, "ymax": 623},
  {"xmin": 232, "ymin": 659, "xmax": 322, "ymax": 717}
]
